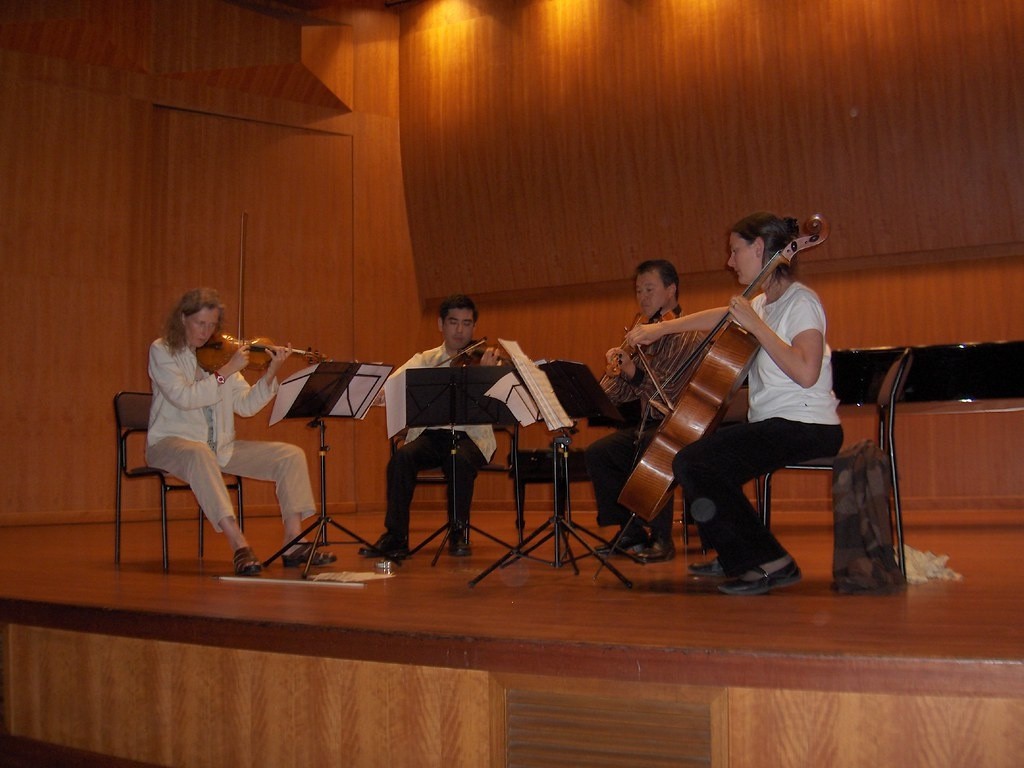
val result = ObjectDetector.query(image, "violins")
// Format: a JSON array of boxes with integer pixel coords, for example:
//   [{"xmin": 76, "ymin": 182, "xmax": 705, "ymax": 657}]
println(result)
[
  {"xmin": 605, "ymin": 310, "xmax": 677, "ymax": 379},
  {"xmin": 449, "ymin": 340, "xmax": 512, "ymax": 367},
  {"xmin": 196, "ymin": 327, "xmax": 334, "ymax": 374}
]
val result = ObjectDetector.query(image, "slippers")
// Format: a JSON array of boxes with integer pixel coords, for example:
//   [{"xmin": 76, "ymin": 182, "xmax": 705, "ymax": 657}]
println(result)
[
  {"xmin": 234, "ymin": 547, "xmax": 263, "ymax": 574},
  {"xmin": 282, "ymin": 541, "xmax": 338, "ymax": 567}
]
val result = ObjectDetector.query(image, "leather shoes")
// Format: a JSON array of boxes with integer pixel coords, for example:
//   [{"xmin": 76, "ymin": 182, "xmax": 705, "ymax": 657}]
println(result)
[
  {"xmin": 448, "ymin": 530, "xmax": 471, "ymax": 556},
  {"xmin": 594, "ymin": 529, "xmax": 648, "ymax": 556},
  {"xmin": 635, "ymin": 534, "xmax": 674, "ymax": 562},
  {"xmin": 718, "ymin": 557, "xmax": 803, "ymax": 595},
  {"xmin": 690, "ymin": 558, "xmax": 730, "ymax": 577},
  {"xmin": 358, "ymin": 533, "xmax": 410, "ymax": 557}
]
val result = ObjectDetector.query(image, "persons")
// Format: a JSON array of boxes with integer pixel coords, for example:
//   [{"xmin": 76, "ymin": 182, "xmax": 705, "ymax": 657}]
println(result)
[
  {"xmin": 585, "ymin": 261, "xmax": 711, "ymax": 563},
  {"xmin": 358, "ymin": 293, "xmax": 502, "ymax": 557},
  {"xmin": 145, "ymin": 286, "xmax": 338, "ymax": 574},
  {"xmin": 625, "ymin": 211, "xmax": 844, "ymax": 595}
]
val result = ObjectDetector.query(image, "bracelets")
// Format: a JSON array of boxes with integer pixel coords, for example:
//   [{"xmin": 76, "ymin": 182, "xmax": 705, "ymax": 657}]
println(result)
[{"xmin": 733, "ymin": 303, "xmax": 738, "ymax": 308}]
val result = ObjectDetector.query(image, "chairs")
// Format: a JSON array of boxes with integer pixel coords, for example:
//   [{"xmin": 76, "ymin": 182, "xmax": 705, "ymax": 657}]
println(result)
[
  {"xmin": 114, "ymin": 391, "xmax": 242, "ymax": 571},
  {"xmin": 682, "ymin": 346, "xmax": 911, "ymax": 582},
  {"xmin": 392, "ymin": 422, "xmax": 594, "ymax": 545}
]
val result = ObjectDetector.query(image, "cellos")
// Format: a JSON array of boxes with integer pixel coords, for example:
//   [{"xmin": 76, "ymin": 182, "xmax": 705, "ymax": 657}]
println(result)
[{"xmin": 593, "ymin": 212, "xmax": 833, "ymax": 582}]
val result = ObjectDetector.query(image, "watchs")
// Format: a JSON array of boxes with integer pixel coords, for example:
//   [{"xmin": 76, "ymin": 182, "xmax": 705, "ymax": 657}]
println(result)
[{"xmin": 215, "ymin": 372, "xmax": 225, "ymax": 385}]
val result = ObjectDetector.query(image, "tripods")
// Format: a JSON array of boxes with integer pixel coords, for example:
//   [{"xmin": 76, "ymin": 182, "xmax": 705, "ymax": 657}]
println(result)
[
  {"xmin": 257, "ymin": 361, "xmax": 403, "ymax": 579},
  {"xmin": 404, "ymin": 360, "xmax": 649, "ymax": 590}
]
